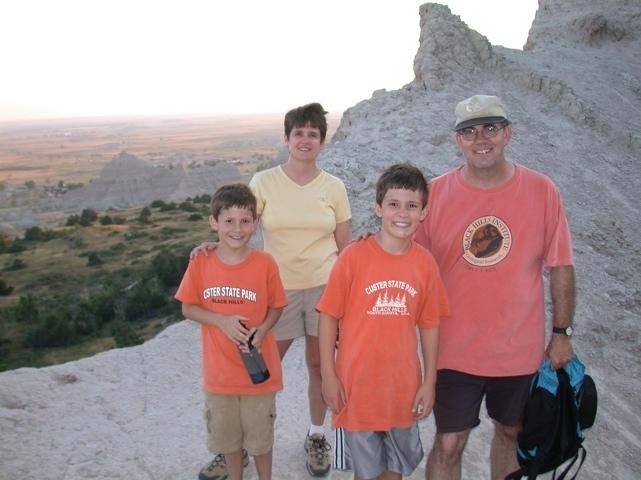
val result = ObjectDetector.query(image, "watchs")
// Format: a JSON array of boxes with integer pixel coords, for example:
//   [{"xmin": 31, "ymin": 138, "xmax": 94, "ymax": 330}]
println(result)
[{"xmin": 552, "ymin": 325, "xmax": 574, "ymax": 337}]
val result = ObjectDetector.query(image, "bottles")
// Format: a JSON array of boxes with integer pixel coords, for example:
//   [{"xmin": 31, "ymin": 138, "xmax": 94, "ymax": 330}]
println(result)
[{"xmin": 234, "ymin": 320, "xmax": 270, "ymax": 385}]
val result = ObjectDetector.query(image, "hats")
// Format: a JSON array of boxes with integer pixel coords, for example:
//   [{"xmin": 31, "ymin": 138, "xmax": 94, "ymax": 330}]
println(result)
[{"xmin": 454, "ymin": 95, "xmax": 511, "ymax": 132}]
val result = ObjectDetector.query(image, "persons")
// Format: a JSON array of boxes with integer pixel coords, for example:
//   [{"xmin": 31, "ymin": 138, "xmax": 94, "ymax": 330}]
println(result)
[
  {"xmin": 314, "ymin": 163, "xmax": 448, "ymax": 480},
  {"xmin": 187, "ymin": 105, "xmax": 355, "ymax": 480},
  {"xmin": 349, "ymin": 96, "xmax": 575, "ymax": 480},
  {"xmin": 174, "ymin": 182, "xmax": 291, "ymax": 480}
]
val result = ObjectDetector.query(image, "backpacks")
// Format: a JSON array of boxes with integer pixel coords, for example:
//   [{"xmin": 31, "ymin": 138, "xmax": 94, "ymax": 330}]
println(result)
[{"xmin": 505, "ymin": 355, "xmax": 597, "ymax": 480}]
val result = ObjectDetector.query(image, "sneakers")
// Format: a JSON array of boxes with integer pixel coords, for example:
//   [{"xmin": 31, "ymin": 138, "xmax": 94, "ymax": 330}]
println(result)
[
  {"xmin": 199, "ymin": 448, "xmax": 249, "ymax": 480},
  {"xmin": 305, "ymin": 429, "xmax": 331, "ymax": 477}
]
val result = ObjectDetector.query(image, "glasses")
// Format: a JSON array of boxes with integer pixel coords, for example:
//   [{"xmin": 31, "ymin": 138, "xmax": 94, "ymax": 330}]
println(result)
[{"xmin": 457, "ymin": 124, "xmax": 508, "ymax": 139}]
